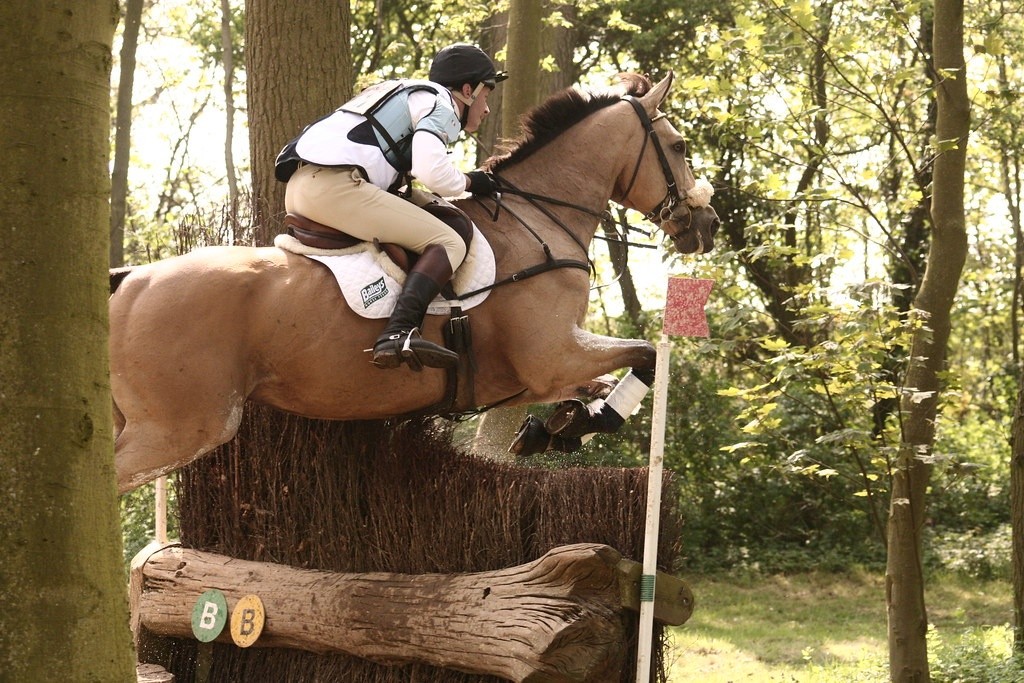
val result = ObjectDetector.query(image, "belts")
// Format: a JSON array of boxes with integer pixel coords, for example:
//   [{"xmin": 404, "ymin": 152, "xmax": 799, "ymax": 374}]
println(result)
[{"xmin": 292, "ymin": 161, "xmax": 308, "ymax": 172}]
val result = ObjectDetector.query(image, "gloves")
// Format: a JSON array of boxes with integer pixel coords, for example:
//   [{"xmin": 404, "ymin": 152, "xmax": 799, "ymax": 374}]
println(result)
[{"xmin": 464, "ymin": 171, "xmax": 498, "ymax": 196}]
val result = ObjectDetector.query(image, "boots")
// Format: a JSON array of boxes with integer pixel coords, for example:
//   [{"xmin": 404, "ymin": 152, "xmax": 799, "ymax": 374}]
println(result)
[{"xmin": 374, "ymin": 272, "xmax": 459, "ymax": 370}]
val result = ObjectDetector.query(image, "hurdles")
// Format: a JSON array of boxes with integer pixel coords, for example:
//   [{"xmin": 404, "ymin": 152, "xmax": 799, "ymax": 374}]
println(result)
[{"xmin": 128, "ymin": 338, "xmax": 672, "ymax": 683}]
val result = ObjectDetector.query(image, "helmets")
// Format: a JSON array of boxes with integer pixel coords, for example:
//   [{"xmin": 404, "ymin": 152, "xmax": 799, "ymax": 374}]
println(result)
[{"xmin": 428, "ymin": 43, "xmax": 508, "ymax": 86}]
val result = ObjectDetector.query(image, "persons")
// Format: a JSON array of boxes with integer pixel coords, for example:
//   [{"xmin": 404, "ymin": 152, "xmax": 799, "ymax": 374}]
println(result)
[{"xmin": 273, "ymin": 43, "xmax": 508, "ymax": 369}]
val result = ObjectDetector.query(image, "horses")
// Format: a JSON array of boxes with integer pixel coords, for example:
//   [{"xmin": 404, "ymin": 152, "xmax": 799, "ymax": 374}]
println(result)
[{"xmin": 107, "ymin": 69, "xmax": 723, "ymax": 497}]
